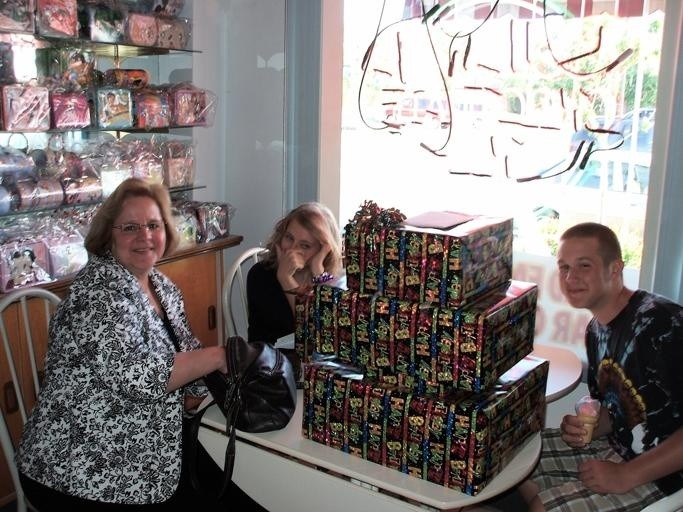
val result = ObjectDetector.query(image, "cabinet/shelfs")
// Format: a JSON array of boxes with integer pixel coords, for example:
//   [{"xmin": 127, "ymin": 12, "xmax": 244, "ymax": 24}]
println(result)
[{"xmin": 0, "ymin": 0, "xmax": 243, "ymax": 508}]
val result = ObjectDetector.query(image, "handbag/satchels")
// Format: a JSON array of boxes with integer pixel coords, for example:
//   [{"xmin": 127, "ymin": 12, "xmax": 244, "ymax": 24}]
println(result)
[{"xmin": 203, "ymin": 336, "xmax": 299, "ymax": 433}]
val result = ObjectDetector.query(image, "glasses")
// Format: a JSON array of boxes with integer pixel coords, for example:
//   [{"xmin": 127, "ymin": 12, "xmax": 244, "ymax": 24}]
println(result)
[{"xmin": 104, "ymin": 220, "xmax": 163, "ymax": 235}]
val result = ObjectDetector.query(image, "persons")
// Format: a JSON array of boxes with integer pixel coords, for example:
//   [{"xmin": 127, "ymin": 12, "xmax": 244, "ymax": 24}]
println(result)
[
  {"xmin": 103, "ymin": 94, "xmax": 119, "ymax": 119},
  {"xmin": 6, "ymin": 250, "xmax": 38, "ymax": 289},
  {"xmin": 518, "ymin": 221, "xmax": 683, "ymax": 512},
  {"xmin": 247, "ymin": 202, "xmax": 342, "ymax": 370},
  {"xmin": 13, "ymin": 175, "xmax": 227, "ymax": 512}
]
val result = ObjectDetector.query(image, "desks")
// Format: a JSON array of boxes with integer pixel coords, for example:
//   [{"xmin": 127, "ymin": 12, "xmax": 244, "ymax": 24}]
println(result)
[
  {"xmin": 534, "ymin": 345, "xmax": 583, "ymax": 404},
  {"xmin": 183, "ymin": 389, "xmax": 542, "ymax": 511}
]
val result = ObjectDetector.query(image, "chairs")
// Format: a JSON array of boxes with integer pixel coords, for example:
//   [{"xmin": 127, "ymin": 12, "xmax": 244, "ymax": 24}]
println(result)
[
  {"xmin": 222, "ymin": 247, "xmax": 271, "ymax": 337},
  {"xmin": 0, "ymin": 286, "xmax": 63, "ymax": 512}
]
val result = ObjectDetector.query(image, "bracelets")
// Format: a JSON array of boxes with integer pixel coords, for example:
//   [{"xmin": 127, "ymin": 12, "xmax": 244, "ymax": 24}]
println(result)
[
  {"xmin": 283, "ymin": 287, "xmax": 299, "ymax": 295},
  {"xmin": 311, "ymin": 272, "xmax": 333, "ymax": 284}
]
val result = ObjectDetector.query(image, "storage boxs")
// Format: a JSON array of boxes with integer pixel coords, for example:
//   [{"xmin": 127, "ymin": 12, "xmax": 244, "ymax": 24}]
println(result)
[
  {"xmin": 294, "ymin": 280, "xmax": 539, "ymax": 394},
  {"xmin": 345, "ymin": 200, "xmax": 513, "ymax": 307},
  {"xmin": 301, "ymin": 358, "xmax": 550, "ymax": 496}
]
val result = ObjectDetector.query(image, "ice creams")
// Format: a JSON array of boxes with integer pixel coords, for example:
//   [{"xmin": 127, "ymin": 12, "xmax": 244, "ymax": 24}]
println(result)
[{"xmin": 574, "ymin": 395, "xmax": 602, "ymax": 444}]
